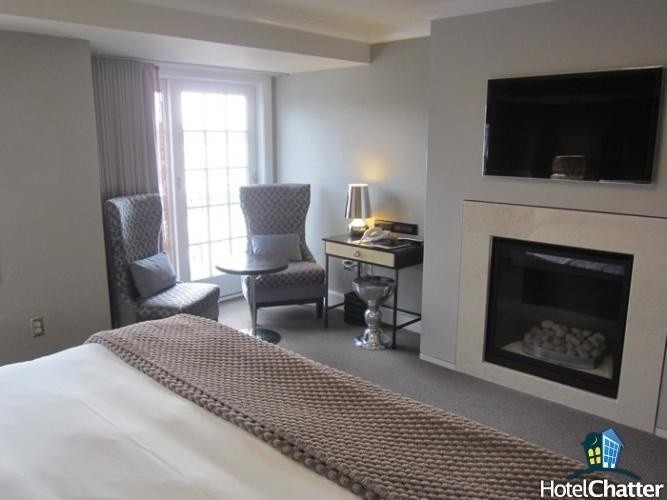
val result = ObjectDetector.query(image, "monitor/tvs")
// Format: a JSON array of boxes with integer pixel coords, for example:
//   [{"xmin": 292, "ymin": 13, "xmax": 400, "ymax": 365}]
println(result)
[{"xmin": 481, "ymin": 65, "xmax": 666, "ymax": 187}]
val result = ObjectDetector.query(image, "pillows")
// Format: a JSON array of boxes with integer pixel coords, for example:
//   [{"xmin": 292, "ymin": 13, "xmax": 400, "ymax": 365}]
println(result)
[
  {"xmin": 127, "ymin": 250, "xmax": 179, "ymax": 299},
  {"xmin": 248, "ymin": 231, "xmax": 304, "ymax": 263}
]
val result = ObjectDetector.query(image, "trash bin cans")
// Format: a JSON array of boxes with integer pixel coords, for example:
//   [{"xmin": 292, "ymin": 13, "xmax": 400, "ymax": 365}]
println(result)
[{"xmin": 344, "ymin": 292, "xmax": 366, "ymax": 326}]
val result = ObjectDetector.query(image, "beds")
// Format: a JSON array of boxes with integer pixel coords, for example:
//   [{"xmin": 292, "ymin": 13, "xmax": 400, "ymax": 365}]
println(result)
[
  {"xmin": 215, "ymin": 258, "xmax": 290, "ymax": 344},
  {"xmin": 0, "ymin": 312, "xmax": 632, "ymax": 497}
]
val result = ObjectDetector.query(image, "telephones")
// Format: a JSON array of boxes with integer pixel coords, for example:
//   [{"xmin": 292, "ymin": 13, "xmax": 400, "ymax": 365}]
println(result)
[{"xmin": 363, "ymin": 227, "xmax": 390, "ymax": 241}]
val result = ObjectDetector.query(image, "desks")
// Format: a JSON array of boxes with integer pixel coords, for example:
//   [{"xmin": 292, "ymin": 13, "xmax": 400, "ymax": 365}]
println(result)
[{"xmin": 322, "ymin": 234, "xmax": 424, "ymax": 350}]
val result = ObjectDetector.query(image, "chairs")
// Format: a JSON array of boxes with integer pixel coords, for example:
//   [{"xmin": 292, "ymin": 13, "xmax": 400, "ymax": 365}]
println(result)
[
  {"xmin": 105, "ymin": 193, "xmax": 220, "ymax": 328},
  {"xmin": 236, "ymin": 184, "xmax": 326, "ymax": 322}
]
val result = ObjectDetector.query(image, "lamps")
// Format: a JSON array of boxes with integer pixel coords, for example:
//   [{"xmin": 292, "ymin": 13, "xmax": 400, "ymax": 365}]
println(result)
[{"xmin": 344, "ymin": 184, "xmax": 372, "ymax": 238}]
